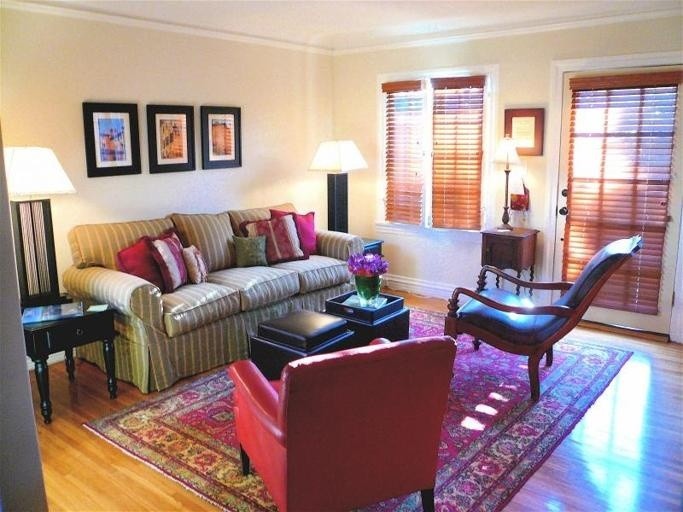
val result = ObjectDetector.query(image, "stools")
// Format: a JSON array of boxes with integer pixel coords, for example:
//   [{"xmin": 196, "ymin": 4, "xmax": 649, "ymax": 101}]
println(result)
[{"xmin": 250, "ymin": 310, "xmax": 354, "ymax": 379}]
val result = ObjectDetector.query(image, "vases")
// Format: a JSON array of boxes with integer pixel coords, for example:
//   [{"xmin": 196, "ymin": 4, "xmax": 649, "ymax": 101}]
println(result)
[{"xmin": 353, "ymin": 276, "xmax": 380, "ymax": 303}]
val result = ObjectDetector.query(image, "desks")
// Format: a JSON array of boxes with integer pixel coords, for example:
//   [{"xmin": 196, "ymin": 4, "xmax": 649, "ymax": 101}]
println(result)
[
  {"xmin": 477, "ymin": 229, "xmax": 536, "ymax": 296},
  {"xmin": 363, "ymin": 238, "xmax": 384, "ymax": 257},
  {"xmin": 18, "ymin": 294, "xmax": 117, "ymax": 424}
]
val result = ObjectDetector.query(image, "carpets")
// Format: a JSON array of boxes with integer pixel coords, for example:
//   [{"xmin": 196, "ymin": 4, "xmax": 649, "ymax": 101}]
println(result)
[{"xmin": 82, "ymin": 308, "xmax": 634, "ymax": 510}]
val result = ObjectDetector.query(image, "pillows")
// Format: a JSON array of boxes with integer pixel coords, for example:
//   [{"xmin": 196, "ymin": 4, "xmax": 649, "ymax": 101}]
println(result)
[
  {"xmin": 239, "ymin": 213, "xmax": 309, "ymax": 262},
  {"xmin": 266, "ymin": 208, "xmax": 317, "ymax": 255},
  {"xmin": 230, "ymin": 233, "xmax": 269, "ymax": 268},
  {"xmin": 182, "ymin": 246, "xmax": 210, "ymax": 283},
  {"xmin": 147, "ymin": 229, "xmax": 186, "ymax": 291},
  {"xmin": 116, "ymin": 234, "xmax": 163, "ymax": 290}
]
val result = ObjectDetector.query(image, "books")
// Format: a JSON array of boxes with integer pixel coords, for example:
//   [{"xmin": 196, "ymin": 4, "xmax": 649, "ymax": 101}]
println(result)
[{"xmin": 21, "ymin": 301, "xmax": 84, "ymax": 324}]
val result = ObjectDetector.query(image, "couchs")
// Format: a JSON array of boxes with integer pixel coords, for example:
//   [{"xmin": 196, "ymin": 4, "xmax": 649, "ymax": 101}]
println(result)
[{"xmin": 61, "ymin": 202, "xmax": 364, "ymax": 392}]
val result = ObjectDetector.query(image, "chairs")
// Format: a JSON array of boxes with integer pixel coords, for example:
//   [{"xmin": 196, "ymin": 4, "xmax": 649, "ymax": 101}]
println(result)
[
  {"xmin": 228, "ymin": 334, "xmax": 457, "ymax": 512},
  {"xmin": 442, "ymin": 236, "xmax": 642, "ymax": 401}
]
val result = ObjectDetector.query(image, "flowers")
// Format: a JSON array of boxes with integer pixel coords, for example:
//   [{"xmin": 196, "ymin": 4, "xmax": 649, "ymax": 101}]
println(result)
[{"xmin": 345, "ymin": 252, "xmax": 388, "ymax": 276}]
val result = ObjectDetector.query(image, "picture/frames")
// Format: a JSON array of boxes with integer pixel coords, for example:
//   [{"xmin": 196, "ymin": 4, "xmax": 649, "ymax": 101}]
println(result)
[
  {"xmin": 82, "ymin": 102, "xmax": 141, "ymax": 179},
  {"xmin": 144, "ymin": 104, "xmax": 195, "ymax": 174},
  {"xmin": 200, "ymin": 106, "xmax": 242, "ymax": 171},
  {"xmin": 502, "ymin": 109, "xmax": 544, "ymax": 156}
]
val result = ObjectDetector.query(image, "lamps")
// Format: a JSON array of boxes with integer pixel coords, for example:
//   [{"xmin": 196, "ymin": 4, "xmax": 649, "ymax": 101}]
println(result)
[
  {"xmin": 3, "ymin": 147, "xmax": 73, "ymax": 316},
  {"xmin": 491, "ymin": 139, "xmax": 521, "ymax": 230},
  {"xmin": 307, "ymin": 140, "xmax": 370, "ymax": 234}
]
